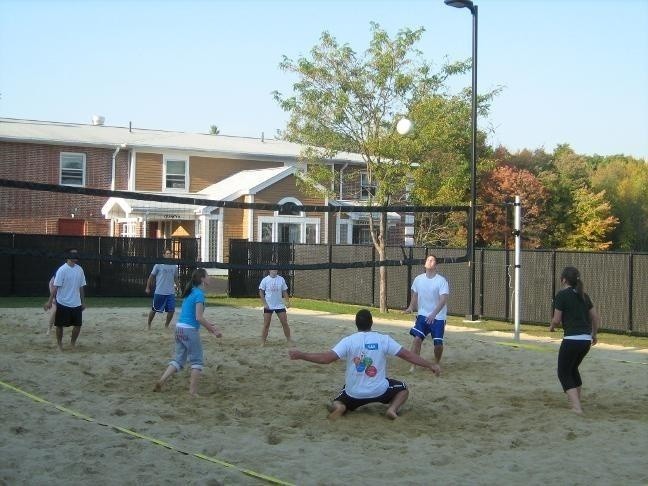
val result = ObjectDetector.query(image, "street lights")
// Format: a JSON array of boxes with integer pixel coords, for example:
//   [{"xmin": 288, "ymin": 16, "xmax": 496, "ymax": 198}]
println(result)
[{"xmin": 440, "ymin": 0, "xmax": 481, "ymax": 322}]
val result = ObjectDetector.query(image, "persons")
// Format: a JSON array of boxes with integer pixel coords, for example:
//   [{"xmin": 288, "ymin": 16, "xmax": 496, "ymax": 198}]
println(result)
[
  {"xmin": 402, "ymin": 255, "xmax": 449, "ymax": 374},
  {"xmin": 550, "ymin": 266, "xmax": 598, "ymax": 415},
  {"xmin": 44, "ymin": 249, "xmax": 87, "ymax": 353},
  {"xmin": 145, "ymin": 249, "xmax": 181, "ymax": 332},
  {"xmin": 258, "ymin": 264, "xmax": 296, "ymax": 347},
  {"xmin": 289, "ymin": 310, "xmax": 440, "ymax": 421},
  {"xmin": 153, "ymin": 268, "xmax": 222, "ymax": 399},
  {"xmin": 47, "ymin": 267, "xmax": 58, "ymax": 335}
]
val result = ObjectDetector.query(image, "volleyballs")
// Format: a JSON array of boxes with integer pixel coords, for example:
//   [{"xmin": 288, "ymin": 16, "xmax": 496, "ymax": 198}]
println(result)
[{"xmin": 398, "ymin": 119, "xmax": 412, "ymax": 135}]
